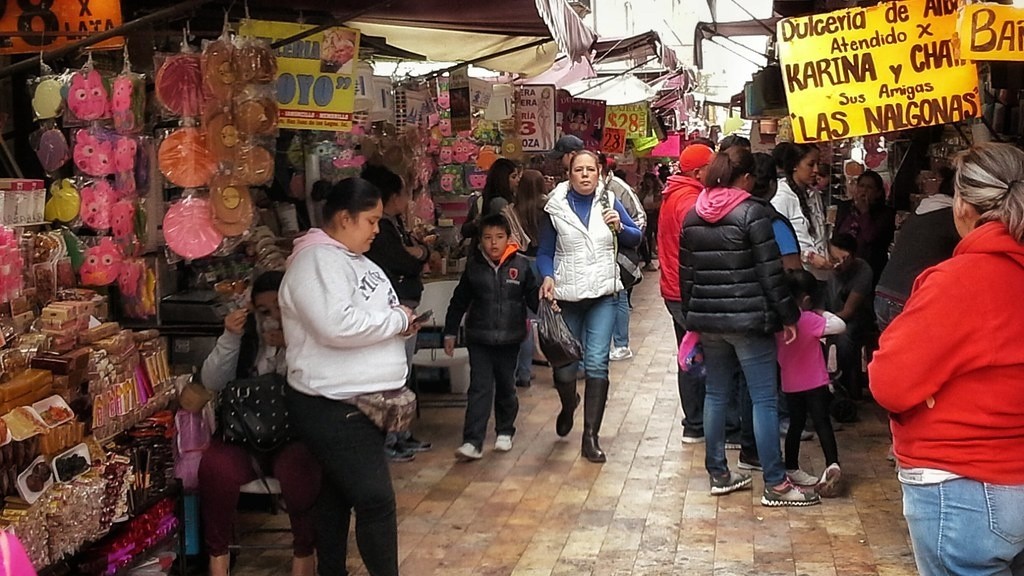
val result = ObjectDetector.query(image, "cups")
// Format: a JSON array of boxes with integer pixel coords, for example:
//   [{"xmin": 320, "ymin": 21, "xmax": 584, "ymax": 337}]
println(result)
[
  {"xmin": 180, "ymin": 383, "xmax": 211, "ymax": 410},
  {"xmin": 971, "ymin": 122, "xmax": 991, "ymax": 143},
  {"xmin": 760, "ymin": 119, "xmax": 778, "ymax": 132}
]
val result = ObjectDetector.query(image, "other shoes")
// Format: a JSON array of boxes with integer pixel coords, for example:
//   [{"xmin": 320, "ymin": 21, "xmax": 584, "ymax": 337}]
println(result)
[
  {"xmin": 829, "ymin": 415, "xmax": 842, "ymax": 430},
  {"xmin": 886, "ymin": 444, "xmax": 896, "ymax": 461},
  {"xmin": 515, "ymin": 378, "xmax": 531, "ymax": 389},
  {"xmin": 780, "ymin": 418, "xmax": 814, "ymax": 441},
  {"xmin": 643, "ymin": 263, "xmax": 657, "ymax": 272},
  {"xmin": 397, "ymin": 433, "xmax": 432, "ymax": 450},
  {"xmin": 533, "ymin": 358, "xmax": 551, "ymax": 367},
  {"xmin": 384, "ymin": 444, "xmax": 416, "ymax": 462}
]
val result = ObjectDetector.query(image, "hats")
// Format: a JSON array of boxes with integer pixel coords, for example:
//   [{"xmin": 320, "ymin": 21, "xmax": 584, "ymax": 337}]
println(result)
[
  {"xmin": 550, "ymin": 135, "xmax": 585, "ymax": 160},
  {"xmin": 680, "ymin": 144, "xmax": 717, "ymax": 173}
]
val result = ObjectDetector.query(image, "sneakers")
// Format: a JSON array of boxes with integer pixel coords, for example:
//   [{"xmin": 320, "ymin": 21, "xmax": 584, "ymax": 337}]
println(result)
[
  {"xmin": 786, "ymin": 466, "xmax": 820, "ymax": 486},
  {"xmin": 681, "ymin": 426, "xmax": 706, "ymax": 442},
  {"xmin": 608, "ymin": 347, "xmax": 633, "ymax": 361},
  {"xmin": 739, "ymin": 449, "xmax": 763, "ymax": 470},
  {"xmin": 494, "ymin": 433, "xmax": 513, "ymax": 451},
  {"xmin": 724, "ymin": 429, "xmax": 742, "ymax": 448},
  {"xmin": 814, "ymin": 462, "xmax": 842, "ymax": 497},
  {"xmin": 761, "ymin": 480, "xmax": 821, "ymax": 506},
  {"xmin": 710, "ymin": 471, "xmax": 754, "ymax": 494},
  {"xmin": 454, "ymin": 444, "xmax": 483, "ymax": 460}
]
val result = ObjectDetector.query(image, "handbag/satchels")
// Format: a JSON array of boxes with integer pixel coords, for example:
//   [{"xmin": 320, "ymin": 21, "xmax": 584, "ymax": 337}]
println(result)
[
  {"xmin": 214, "ymin": 373, "xmax": 290, "ymax": 445},
  {"xmin": 536, "ymin": 297, "xmax": 584, "ymax": 370},
  {"xmin": 355, "ymin": 386, "xmax": 417, "ymax": 431}
]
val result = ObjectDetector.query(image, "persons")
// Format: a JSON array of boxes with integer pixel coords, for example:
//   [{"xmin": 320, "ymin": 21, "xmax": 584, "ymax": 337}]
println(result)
[
  {"xmin": 549, "ymin": 135, "xmax": 584, "ymax": 171},
  {"xmin": 827, "ymin": 232, "xmax": 872, "ymax": 360},
  {"xmin": 598, "ymin": 153, "xmax": 646, "ymax": 361},
  {"xmin": 358, "ymin": 163, "xmax": 432, "ymax": 462},
  {"xmin": 773, "ymin": 269, "xmax": 846, "ymax": 495},
  {"xmin": 441, "ymin": 213, "xmax": 562, "ymax": 459},
  {"xmin": 535, "ymin": 150, "xmax": 642, "ymax": 460},
  {"xmin": 771, "ymin": 143, "xmax": 842, "ymax": 440},
  {"xmin": 738, "ymin": 151, "xmax": 801, "ymax": 470},
  {"xmin": 199, "ymin": 270, "xmax": 322, "ymax": 576},
  {"xmin": 679, "ymin": 146, "xmax": 821, "ymax": 506},
  {"xmin": 832, "ymin": 170, "xmax": 898, "ymax": 283},
  {"xmin": 868, "ymin": 143, "xmax": 1024, "ymax": 576},
  {"xmin": 872, "ymin": 165, "xmax": 962, "ymax": 331},
  {"xmin": 501, "ymin": 170, "xmax": 548, "ymax": 387},
  {"xmin": 460, "ymin": 157, "xmax": 520, "ymax": 240},
  {"xmin": 657, "ymin": 143, "xmax": 742, "ymax": 450},
  {"xmin": 277, "ymin": 177, "xmax": 422, "ymax": 576},
  {"xmin": 637, "ymin": 173, "xmax": 664, "ymax": 257}
]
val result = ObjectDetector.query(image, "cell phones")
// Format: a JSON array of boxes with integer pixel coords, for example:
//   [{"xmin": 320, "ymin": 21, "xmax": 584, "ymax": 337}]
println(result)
[{"xmin": 409, "ymin": 310, "xmax": 433, "ymax": 327}]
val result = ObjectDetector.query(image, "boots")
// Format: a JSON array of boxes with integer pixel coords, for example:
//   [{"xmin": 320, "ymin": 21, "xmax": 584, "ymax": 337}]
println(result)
[
  {"xmin": 552, "ymin": 378, "xmax": 582, "ymax": 436},
  {"xmin": 582, "ymin": 377, "xmax": 610, "ymax": 463}
]
